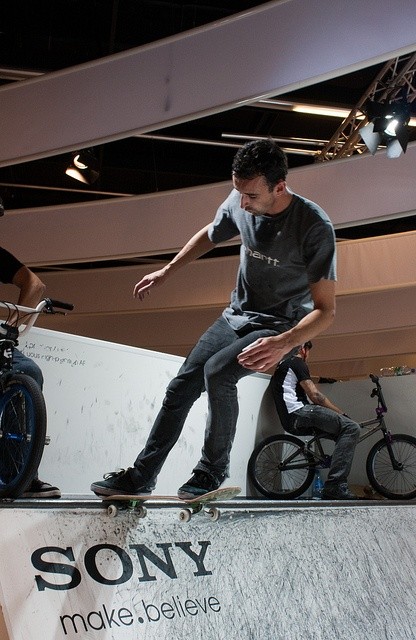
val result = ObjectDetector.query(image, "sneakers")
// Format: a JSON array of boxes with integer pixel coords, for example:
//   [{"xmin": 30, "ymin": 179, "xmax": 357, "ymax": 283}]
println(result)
[
  {"xmin": 90, "ymin": 467, "xmax": 151, "ymax": 496},
  {"xmin": 322, "ymin": 482, "xmax": 357, "ymax": 500},
  {"xmin": 22, "ymin": 479, "xmax": 61, "ymax": 498},
  {"xmin": 177, "ymin": 463, "xmax": 229, "ymax": 500}
]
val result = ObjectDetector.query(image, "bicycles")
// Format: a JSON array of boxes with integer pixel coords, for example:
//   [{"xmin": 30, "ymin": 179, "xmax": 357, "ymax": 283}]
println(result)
[
  {"xmin": 0, "ymin": 297, "xmax": 74, "ymax": 503},
  {"xmin": 248, "ymin": 372, "xmax": 416, "ymax": 499}
]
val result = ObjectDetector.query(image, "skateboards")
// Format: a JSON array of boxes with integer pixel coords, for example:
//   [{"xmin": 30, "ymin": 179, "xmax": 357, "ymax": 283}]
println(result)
[{"xmin": 3, "ymin": 487, "xmax": 240, "ymax": 522}]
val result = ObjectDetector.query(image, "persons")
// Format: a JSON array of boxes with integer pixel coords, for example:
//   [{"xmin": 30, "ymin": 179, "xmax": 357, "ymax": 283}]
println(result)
[
  {"xmin": 91, "ymin": 139, "xmax": 337, "ymax": 497},
  {"xmin": 270, "ymin": 341, "xmax": 361, "ymax": 500},
  {"xmin": 1, "ymin": 247, "xmax": 62, "ymax": 499}
]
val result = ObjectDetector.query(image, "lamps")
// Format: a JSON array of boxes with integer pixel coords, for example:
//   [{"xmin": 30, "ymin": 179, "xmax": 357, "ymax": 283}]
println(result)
[
  {"xmin": 386, "ymin": 134, "xmax": 409, "ymax": 158},
  {"xmin": 358, "ymin": 121, "xmax": 382, "ymax": 157},
  {"xmin": 372, "ymin": 108, "xmax": 411, "ymax": 155},
  {"xmin": 65, "ymin": 152, "xmax": 100, "ymax": 185}
]
